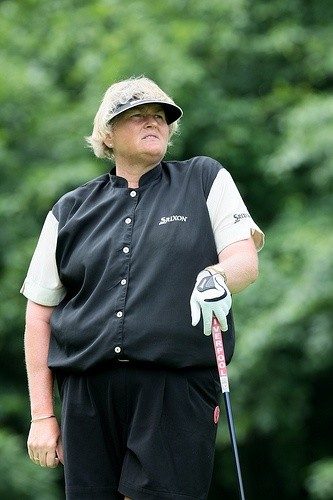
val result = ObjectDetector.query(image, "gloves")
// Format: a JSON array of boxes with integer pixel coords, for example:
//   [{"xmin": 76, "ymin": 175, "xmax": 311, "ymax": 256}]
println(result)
[{"xmin": 189, "ymin": 269, "xmax": 232, "ymax": 336}]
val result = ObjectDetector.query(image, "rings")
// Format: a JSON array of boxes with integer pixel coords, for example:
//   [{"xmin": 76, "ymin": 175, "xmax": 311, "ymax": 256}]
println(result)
[{"xmin": 34, "ymin": 456, "xmax": 39, "ymax": 461}]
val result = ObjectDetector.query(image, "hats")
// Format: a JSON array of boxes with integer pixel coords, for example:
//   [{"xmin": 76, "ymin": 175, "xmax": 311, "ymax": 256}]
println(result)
[{"xmin": 107, "ymin": 96, "xmax": 182, "ymax": 126}]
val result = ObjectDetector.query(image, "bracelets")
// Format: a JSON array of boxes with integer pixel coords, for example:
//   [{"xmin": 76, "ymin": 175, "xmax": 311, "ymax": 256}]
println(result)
[{"xmin": 29, "ymin": 414, "xmax": 57, "ymax": 423}]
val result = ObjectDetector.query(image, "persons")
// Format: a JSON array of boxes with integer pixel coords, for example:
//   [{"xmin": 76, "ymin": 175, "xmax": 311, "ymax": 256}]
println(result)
[{"xmin": 20, "ymin": 76, "xmax": 267, "ymax": 500}]
